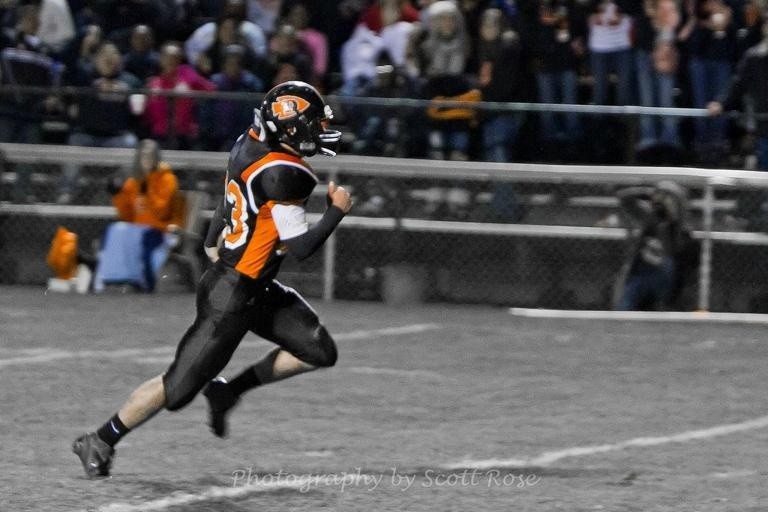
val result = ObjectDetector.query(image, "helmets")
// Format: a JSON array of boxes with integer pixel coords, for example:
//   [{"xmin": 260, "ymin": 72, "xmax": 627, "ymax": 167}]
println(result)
[{"xmin": 256, "ymin": 81, "xmax": 341, "ymax": 159}]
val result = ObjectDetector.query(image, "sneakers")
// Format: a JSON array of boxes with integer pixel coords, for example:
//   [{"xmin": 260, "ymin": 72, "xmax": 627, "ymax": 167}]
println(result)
[
  {"xmin": 72, "ymin": 433, "xmax": 112, "ymax": 481},
  {"xmin": 204, "ymin": 379, "xmax": 238, "ymax": 435}
]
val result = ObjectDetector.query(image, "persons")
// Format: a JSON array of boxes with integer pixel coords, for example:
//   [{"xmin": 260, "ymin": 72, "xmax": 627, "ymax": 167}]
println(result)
[
  {"xmin": 0, "ymin": 0, "xmax": 765, "ymax": 309},
  {"xmin": 72, "ymin": 80, "xmax": 356, "ymax": 480}
]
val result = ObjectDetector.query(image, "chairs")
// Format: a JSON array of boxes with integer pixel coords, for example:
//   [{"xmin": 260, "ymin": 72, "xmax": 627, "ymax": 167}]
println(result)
[{"xmin": 83, "ymin": 185, "xmax": 210, "ymax": 292}]
